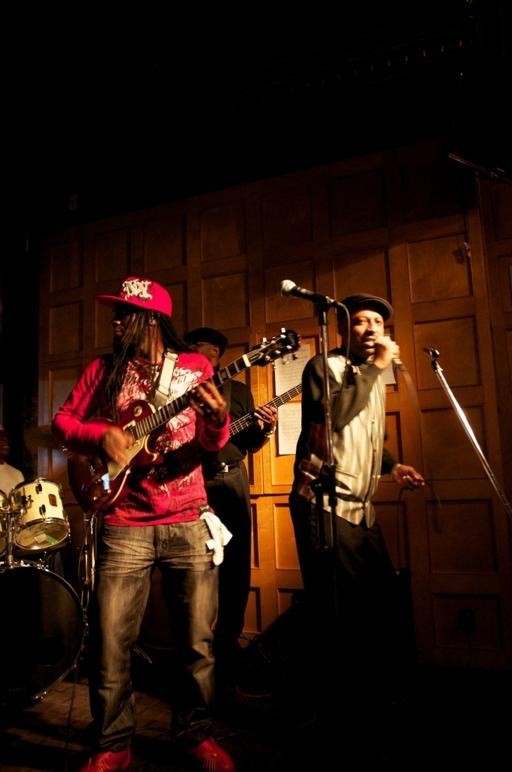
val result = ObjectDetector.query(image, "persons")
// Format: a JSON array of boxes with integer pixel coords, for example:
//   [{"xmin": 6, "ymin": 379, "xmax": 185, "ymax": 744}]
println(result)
[
  {"xmin": 289, "ymin": 292, "xmax": 425, "ymax": 668},
  {"xmin": 0, "ymin": 424, "xmax": 25, "ymax": 507},
  {"xmin": 50, "ymin": 276, "xmax": 234, "ymax": 771},
  {"xmin": 158, "ymin": 327, "xmax": 279, "ymax": 649}
]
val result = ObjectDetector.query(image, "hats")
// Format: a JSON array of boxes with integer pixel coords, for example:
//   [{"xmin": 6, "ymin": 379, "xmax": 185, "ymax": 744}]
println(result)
[
  {"xmin": 336, "ymin": 294, "xmax": 392, "ymax": 324},
  {"xmin": 98, "ymin": 277, "xmax": 172, "ymax": 317},
  {"xmin": 183, "ymin": 327, "xmax": 228, "ymax": 353}
]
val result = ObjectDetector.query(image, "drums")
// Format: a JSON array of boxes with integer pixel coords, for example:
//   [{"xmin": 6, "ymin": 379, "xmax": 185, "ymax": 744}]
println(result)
[
  {"xmin": 9, "ymin": 476, "xmax": 71, "ymax": 550},
  {"xmin": 0, "ymin": 490, "xmax": 8, "ymax": 556},
  {"xmin": 0, "ymin": 560, "xmax": 86, "ymax": 708}
]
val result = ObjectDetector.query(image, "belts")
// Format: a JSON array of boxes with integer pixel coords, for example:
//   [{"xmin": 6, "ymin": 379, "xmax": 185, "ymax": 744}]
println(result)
[{"xmin": 218, "ymin": 461, "xmax": 238, "ymax": 474}]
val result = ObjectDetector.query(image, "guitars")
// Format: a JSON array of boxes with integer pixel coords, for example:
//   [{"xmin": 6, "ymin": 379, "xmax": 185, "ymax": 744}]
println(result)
[{"xmin": 67, "ymin": 329, "xmax": 300, "ymax": 517}]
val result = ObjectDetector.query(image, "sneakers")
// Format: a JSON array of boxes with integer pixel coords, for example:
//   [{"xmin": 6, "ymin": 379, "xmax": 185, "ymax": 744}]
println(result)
[
  {"xmin": 84, "ymin": 750, "xmax": 132, "ymax": 771},
  {"xmin": 194, "ymin": 739, "xmax": 232, "ymax": 772}
]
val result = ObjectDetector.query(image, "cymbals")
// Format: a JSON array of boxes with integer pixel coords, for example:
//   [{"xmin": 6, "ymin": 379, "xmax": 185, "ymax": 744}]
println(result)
[{"xmin": 24, "ymin": 424, "xmax": 65, "ymax": 452}]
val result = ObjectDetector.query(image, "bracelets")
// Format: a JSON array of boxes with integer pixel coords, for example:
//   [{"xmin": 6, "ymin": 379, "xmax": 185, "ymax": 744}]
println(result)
[{"xmin": 264, "ymin": 426, "xmax": 276, "ymax": 439}]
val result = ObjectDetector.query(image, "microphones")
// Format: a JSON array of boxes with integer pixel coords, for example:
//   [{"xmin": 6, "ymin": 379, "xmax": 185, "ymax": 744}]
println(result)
[
  {"xmin": 374, "ymin": 335, "xmax": 402, "ymax": 369},
  {"xmin": 280, "ymin": 279, "xmax": 339, "ymax": 308}
]
